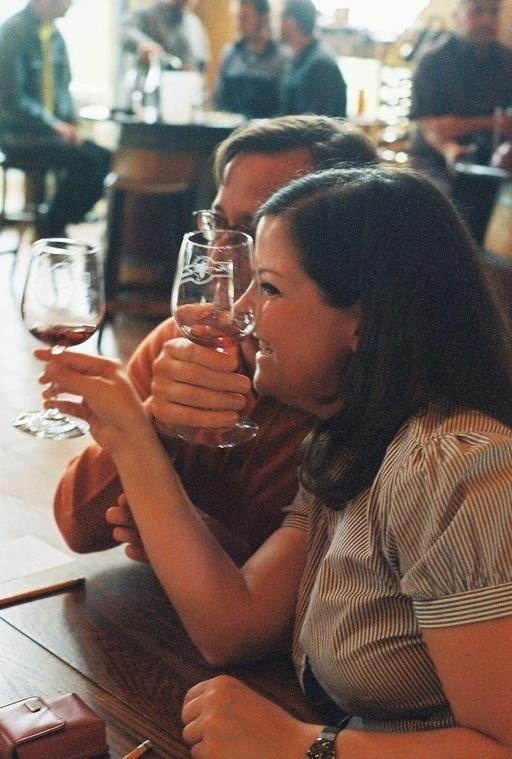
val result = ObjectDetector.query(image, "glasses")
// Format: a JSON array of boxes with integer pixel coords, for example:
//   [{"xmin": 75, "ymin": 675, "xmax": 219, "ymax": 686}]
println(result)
[{"xmin": 194, "ymin": 207, "xmax": 257, "ymax": 260}]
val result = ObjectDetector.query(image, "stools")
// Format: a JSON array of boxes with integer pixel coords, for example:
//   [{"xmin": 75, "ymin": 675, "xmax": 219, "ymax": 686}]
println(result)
[
  {"xmin": 1, "ymin": 159, "xmax": 63, "ymax": 260},
  {"xmin": 97, "ymin": 179, "xmax": 193, "ymax": 356}
]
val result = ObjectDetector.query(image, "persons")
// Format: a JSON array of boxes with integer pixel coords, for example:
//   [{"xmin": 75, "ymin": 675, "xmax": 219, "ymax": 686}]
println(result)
[
  {"xmin": 204, "ymin": 0, "xmax": 284, "ymax": 125},
  {"xmin": 32, "ymin": 164, "xmax": 512, "ymax": 759},
  {"xmin": 249, "ymin": 0, "xmax": 349, "ymax": 120},
  {"xmin": 408, "ymin": 0, "xmax": 512, "ymax": 250},
  {"xmin": 51, "ymin": 111, "xmax": 385, "ymax": 570},
  {"xmin": 0, "ymin": 0, "xmax": 112, "ymax": 245},
  {"xmin": 113, "ymin": 0, "xmax": 205, "ymax": 111}
]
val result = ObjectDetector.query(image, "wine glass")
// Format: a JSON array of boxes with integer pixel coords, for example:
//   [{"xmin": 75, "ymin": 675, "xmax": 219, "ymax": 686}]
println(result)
[
  {"xmin": 12, "ymin": 238, "xmax": 105, "ymax": 443},
  {"xmin": 170, "ymin": 230, "xmax": 262, "ymax": 450}
]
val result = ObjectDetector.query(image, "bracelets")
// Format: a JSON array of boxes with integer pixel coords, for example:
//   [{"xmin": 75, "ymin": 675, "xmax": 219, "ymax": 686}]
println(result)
[{"xmin": 306, "ymin": 725, "xmax": 342, "ymax": 758}]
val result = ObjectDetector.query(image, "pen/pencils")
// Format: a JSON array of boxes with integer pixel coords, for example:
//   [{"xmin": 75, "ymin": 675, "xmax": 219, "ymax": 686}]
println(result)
[
  {"xmin": 0, "ymin": 577, "xmax": 86, "ymax": 606},
  {"xmin": 122, "ymin": 739, "xmax": 154, "ymax": 759}
]
